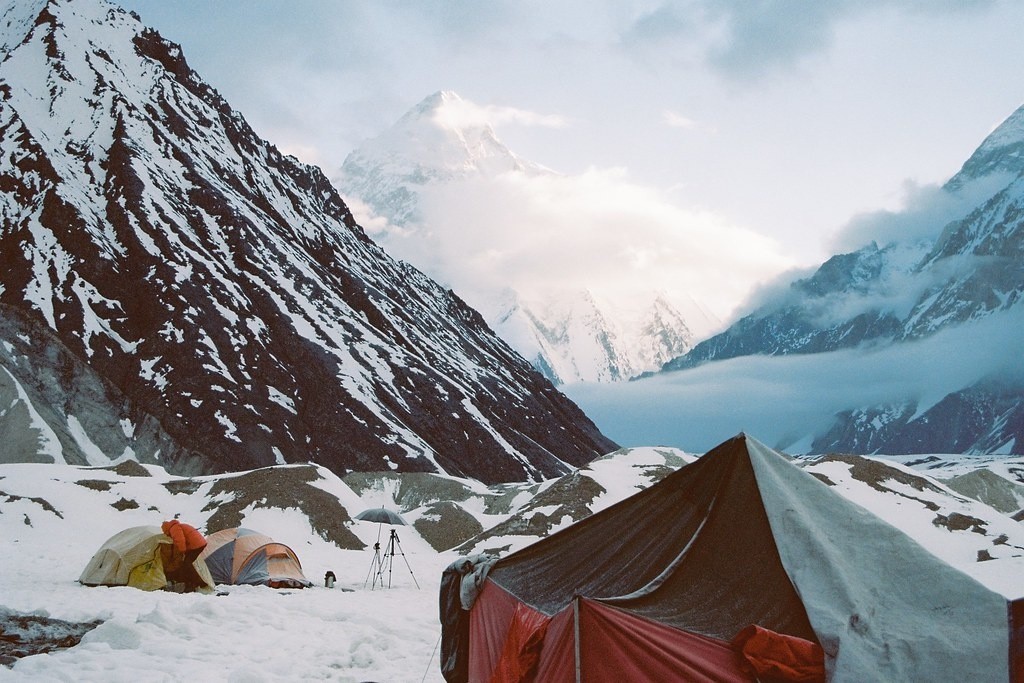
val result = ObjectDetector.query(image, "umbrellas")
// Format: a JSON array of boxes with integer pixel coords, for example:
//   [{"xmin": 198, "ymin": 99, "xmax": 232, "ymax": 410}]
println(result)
[{"xmin": 352, "ymin": 505, "xmax": 408, "ymax": 545}]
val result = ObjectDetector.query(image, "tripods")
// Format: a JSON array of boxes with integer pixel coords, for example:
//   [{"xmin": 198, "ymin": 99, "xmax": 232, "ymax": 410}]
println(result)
[{"xmin": 364, "ymin": 529, "xmax": 420, "ymax": 592}]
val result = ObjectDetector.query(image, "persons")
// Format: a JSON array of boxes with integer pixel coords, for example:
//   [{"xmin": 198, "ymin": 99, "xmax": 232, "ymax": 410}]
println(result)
[{"xmin": 161, "ymin": 521, "xmax": 207, "ymax": 593}]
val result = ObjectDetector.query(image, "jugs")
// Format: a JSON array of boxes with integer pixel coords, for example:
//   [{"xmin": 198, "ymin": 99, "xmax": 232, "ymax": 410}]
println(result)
[{"xmin": 324, "ymin": 571, "xmax": 334, "ymax": 588}]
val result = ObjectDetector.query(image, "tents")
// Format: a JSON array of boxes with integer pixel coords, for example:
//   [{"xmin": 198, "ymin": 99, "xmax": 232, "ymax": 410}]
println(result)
[
  {"xmin": 439, "ymin": 429, "xmax": 1023, "ymax": 683},
  {"xmin": 77, "ymin": 525, "xmax": 216, "ymax": 594},
  {"xmin": 202, "ymin": 528, "xmax": 313, "ymax": 589}
]
